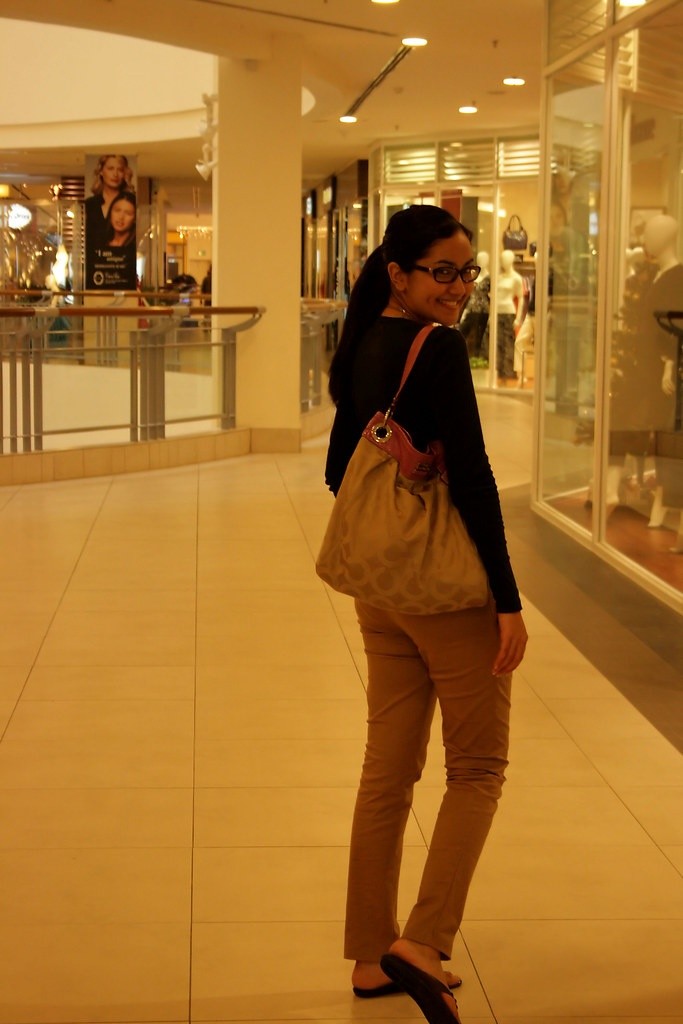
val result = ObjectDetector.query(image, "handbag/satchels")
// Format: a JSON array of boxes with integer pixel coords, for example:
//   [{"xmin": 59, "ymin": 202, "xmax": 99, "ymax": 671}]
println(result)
[
  {"xmin": 316, "ymin": 318, "xmax": 492, "ymax": 614},
  {"xmin": 503, "ymin": 214, "xmax": 529, "ymax": 249}
]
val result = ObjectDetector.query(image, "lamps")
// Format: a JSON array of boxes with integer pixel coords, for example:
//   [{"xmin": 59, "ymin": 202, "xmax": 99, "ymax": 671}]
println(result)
[{"xmin": 194, "ymin": 160, "xmax": 215, "ymax": 180}]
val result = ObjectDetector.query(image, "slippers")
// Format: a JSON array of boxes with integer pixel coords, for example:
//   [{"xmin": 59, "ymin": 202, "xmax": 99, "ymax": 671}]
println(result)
[
  {"xmin": 380, "ymin": 951, "xmax": 460, "ymax": 1022},
  {"xmin": 353, "ymin": 972, "xmax": 462, "ymax": 999}
]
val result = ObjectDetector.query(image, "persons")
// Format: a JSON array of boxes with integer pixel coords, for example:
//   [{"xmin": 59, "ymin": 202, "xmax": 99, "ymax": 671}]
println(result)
[
  {"xmin": 603, "ymin": 215, "xmax": 683, "ymax": 551},
  {"xmin": 322, "ymin": 206, "xmax": 529, "ymax": 1024},
  {"xmin": 85, "ymin": 155, "xmax": 135, "ymax": 248},
  {"xmin": 201, "ymin": 265, "xmax": 213, "ymax": 301},
  {"xmin": 462, "ymin": 250, "xmax": 525, "ymax": 378}
]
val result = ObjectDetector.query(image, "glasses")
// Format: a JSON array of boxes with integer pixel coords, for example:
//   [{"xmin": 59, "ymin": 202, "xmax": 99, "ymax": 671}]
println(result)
[{"xmin": 411, "ymin": 263, "xmax": 484, "ymax": 284}]
